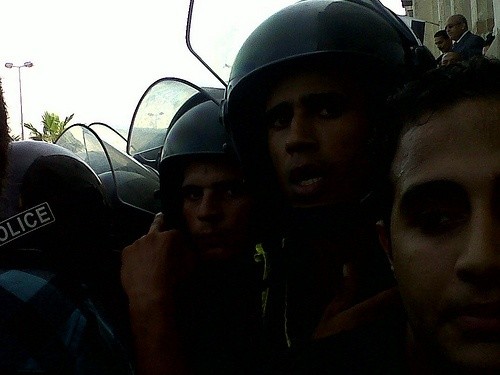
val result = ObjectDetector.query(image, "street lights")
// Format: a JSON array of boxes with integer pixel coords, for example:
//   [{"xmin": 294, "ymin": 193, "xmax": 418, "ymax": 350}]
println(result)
[{"xmin": 4, "ymin": 60, "xmax": 33, "ymax": 140}]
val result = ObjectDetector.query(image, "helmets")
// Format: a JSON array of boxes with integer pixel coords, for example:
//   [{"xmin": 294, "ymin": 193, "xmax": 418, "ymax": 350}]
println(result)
[
  {"xmin": 226, "ymin": 0, "xmax": 405, "ymax": 148},
  {"xmin": 98, "ymin": 172, "xmax": 161, "ymax": 217},
  {"xmin": 159, "ymin": 99, "xmax": 230, "ymax": 225}
]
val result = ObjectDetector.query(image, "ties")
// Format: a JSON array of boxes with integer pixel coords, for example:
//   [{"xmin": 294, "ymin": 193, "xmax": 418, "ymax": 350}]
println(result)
[{"xmin": 453, "ymin": 43, "xmax": 459, "ymax": 49}]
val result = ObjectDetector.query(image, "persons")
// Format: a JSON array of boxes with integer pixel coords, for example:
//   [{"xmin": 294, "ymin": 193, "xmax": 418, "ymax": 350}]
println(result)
[{"xmin": 0, "ymin": 14, "xmax": 500, "ymax": 375}]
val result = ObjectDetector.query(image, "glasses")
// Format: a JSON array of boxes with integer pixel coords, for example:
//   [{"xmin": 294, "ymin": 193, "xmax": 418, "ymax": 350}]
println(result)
[{"xmin": 445, "ymin": 20, "xmax": 463, "ymax": 30}]
